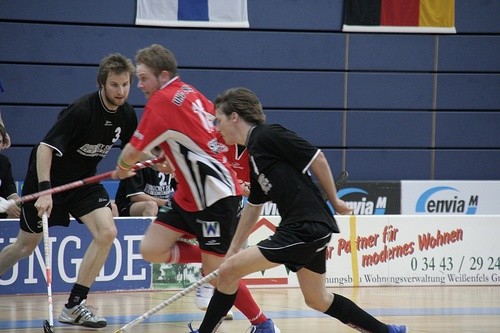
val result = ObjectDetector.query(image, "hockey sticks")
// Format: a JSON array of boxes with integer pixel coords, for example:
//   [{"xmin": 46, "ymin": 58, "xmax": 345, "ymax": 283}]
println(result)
[
  {"xmin": 8, "ymin": 157, "xmax": 165, "ymax": 204},
  {"xmin": 112, "ymin": 269, "xmax": 218, "ymax": 333},
  {"xmin": 42, "ymin": 211, "xmax": 56, "ymax": 333}
]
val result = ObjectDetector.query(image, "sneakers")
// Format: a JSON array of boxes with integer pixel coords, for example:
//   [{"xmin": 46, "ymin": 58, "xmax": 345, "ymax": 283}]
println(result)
[
  {"xmin": 249, "ymin": 315, "xmax": 281, "ymax": 333},
  {"xmin": 57, "ymin": 300, "xmax": 107, "ymax": 329},
  {"xmin": 195, "ymin": 267, "xmax": 212, "ymax": 310}
]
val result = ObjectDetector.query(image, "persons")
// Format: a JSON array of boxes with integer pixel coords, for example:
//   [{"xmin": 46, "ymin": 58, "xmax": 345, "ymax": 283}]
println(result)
[
  {"xmin": 0, "ymin": 122, "xmax": 22, "ymax": 219},
  {"xmin": 112, "ymin": 44, "xmax": 280, "ymax": 333},
  {"xmin": 0, "ymin": 53, "xmax": 166, "ymax": 328},
  {"xmin": 224, "ymin": 136, "xmax": 251, "ymax": 320},
  {"xmin": 114, "ymin": 145, "xmax": 177, "ymax": 215},
  {"xmin": 187, "ymin": 87, "xmax": 406, "ymax": 333}
]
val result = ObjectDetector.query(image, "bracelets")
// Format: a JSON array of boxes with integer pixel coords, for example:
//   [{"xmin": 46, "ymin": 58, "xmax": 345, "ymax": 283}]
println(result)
[
  {"xmin": 39, "ymin": 181, "xmax": 51, "ymax": 192},
  {"xmin": 117, "ymin": 156, "xmax": 132, "ymax": 171}
]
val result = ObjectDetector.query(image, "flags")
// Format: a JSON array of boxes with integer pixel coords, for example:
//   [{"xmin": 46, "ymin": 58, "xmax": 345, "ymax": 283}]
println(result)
[
  {"xmin": 135, "ymin": 0, "xmax": 250, "ymax": 28},
  {"xmin": 341, "ymin": 0, "xmax": 456, "ymax": 33}
]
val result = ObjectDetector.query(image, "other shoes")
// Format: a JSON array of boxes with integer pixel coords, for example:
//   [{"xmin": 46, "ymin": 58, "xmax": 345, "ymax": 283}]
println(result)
[{"xmin": 388, "ymin": 323, "xmax": 408, "ymax": 333}]
